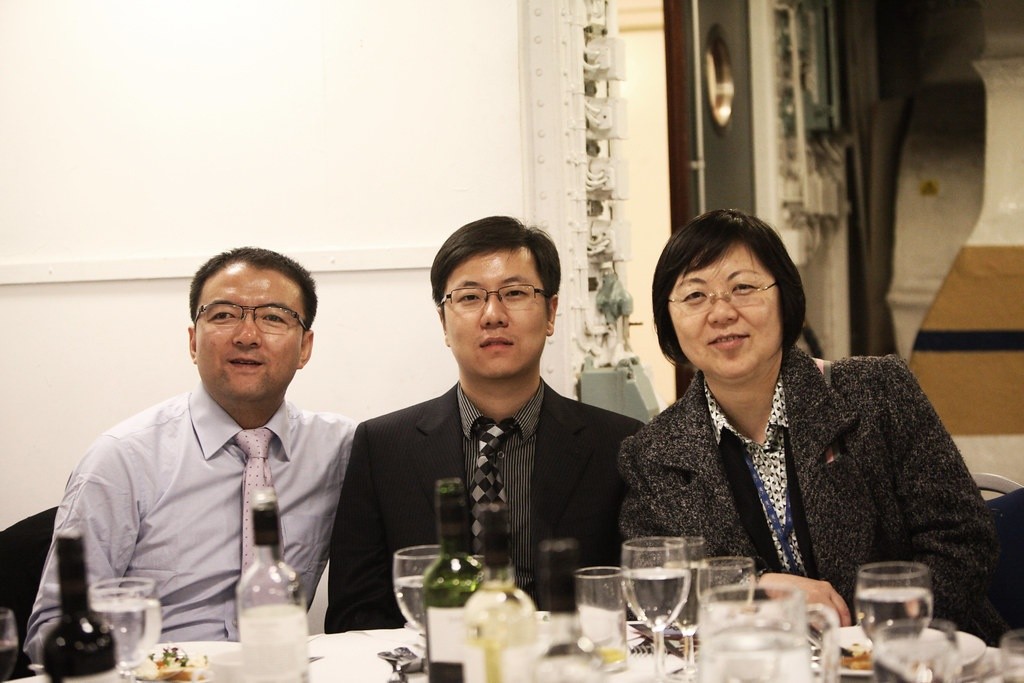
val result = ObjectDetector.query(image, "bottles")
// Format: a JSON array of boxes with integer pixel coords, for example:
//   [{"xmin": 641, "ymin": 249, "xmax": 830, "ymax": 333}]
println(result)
[
  {"xmin": 42, "ymin": 527, "xmax": 125, "ymax": 683},
  {"xmin": 459, "ymin": 500, "xmax": 544, "ymax": 683},
  {"xmin": 421, "ymin": 478, "xmax": 486, "ymax": 683},
  {"xmin": 233, "ymin": 486, "xmax": 312, "ymax": 683}
]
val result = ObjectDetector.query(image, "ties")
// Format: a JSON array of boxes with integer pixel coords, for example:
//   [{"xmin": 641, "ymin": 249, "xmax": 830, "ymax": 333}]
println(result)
[
  {"xmin": 235, "ymin": 429, "xmax": 284, "ymax": 608},
  {"xmin": 471, "ymin": 422, "xmax": 514, "ymax": 585}
]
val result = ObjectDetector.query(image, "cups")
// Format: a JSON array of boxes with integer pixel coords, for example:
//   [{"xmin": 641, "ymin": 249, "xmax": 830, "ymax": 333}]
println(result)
[
  {"xmin": 392, "ymin": 544, "xmax": 441, "ymax": 636},
  {"xmin": 571, "ymin": 566, "xmax": 630, "ymax": 674},
  {"xmin": 695, "ymin": 582, "xmax": 843, "ymax": 682},
  {"xmin": 1000, "ymin": 631, "xmax": 1024, "ymax": 683},
  {"xmin": 853, "ymin": 558, "xmax": 933, "ymax": 646},
  {"xmin": 870, "ymin": 618, "xmax": 962, "ymax": 683}
]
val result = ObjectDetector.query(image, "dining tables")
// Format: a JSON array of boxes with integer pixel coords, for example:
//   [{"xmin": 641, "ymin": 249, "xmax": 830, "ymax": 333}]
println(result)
[{"xmin": 0, "ymin": 537, "xmax": 1024, "ymax": 683}]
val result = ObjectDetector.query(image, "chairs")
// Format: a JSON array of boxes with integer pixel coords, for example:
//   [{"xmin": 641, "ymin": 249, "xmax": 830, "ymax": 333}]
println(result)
[
  {"xmin": 970, "ymin": 471, "xmax": 1024, "ymax": 629},
  {"xmin": 0, "ymin": 504, "xmax": 63, "ymax": 683}
]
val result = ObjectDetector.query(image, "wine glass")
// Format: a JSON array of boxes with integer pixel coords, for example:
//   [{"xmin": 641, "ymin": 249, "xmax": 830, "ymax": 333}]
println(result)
[
  {"xmin": 620, "ymin": 535, "xmax": 758, "ymax": 683},
  {"xmin": 87, "ymin": 576, "xmax": 164, "ymax": 683}
]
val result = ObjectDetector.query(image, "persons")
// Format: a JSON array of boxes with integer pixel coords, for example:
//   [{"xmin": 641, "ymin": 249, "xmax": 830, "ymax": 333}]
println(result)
[
  {"xmin": 22, "ymin": 247, "xmax": 358, "ymax": 665},
  {"xmin": 614, "ymin": 207, "xmax": 1009, "ymax": 648},
  {"xmin": 324, "ymin": 215, "xmax": 646, "ymax": 634}
]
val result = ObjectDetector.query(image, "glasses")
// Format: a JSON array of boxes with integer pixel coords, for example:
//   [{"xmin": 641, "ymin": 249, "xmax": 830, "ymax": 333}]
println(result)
[
  {"xmin": 441, "ymin": 284, "xmax": 545, "ymax": 309},
  {"xmin": 195, "ymin": 302, "xmax": 307, "ymax": 330},
  {"xmin": 668, "ymin": 283, "xmax": 775, "ymax": 302}
]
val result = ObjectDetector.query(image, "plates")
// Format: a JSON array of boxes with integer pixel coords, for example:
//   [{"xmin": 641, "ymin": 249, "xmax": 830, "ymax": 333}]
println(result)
[{"xmin": 839, "ymin": 624, "xmax": 987, "ymax": 677}]
